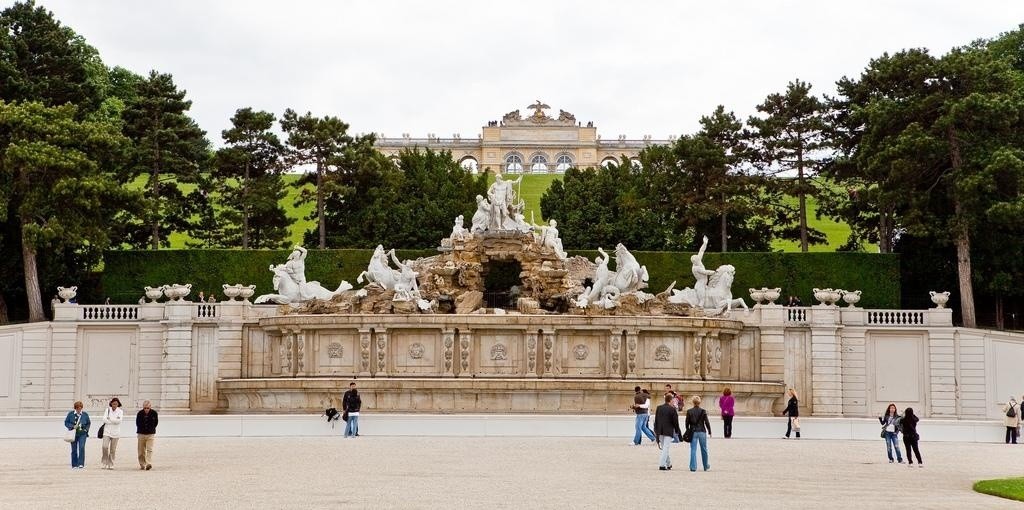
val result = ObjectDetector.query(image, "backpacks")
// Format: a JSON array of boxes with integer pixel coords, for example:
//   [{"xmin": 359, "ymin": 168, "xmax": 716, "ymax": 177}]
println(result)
[
  {"xmin": 1006, "ymin": 402, "xmax": 1016, "ymax": 417},
  {"xmin": 675, "ymin": 393, "xmax": 683, "ymax": 411}
]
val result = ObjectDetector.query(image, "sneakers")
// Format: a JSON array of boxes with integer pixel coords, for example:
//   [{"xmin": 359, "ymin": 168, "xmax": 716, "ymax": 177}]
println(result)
[
  {"xmin": 71, "ymin": 464, "xmax": 152, "ymax": 472},
  {"xmin": 889, "ymin": 460, "xmax": 923, "ymax": 468}
]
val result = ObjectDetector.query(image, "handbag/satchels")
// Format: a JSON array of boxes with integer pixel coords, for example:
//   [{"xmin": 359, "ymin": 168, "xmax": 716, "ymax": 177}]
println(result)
[
  {"xmin": 97, "ymin": 424, "xmax": 104, "ymax": 438},
  {"xmin": 682, "ymin": 428, "xmax": 692, "ymax": 443},
  {"xmin": 62, "ymin": 428, "xmax": 76, "ymax": 442},
  {"xmin": 790, "ymin": 416, "xmax": 800, "ymax": 432},
  {"xmin": 342, "ymin": 408, "xmax": 348, "ymax": 421},
  {"xmin": 880, "ymin": 425, "xmax": 887, "ymax": 438}
]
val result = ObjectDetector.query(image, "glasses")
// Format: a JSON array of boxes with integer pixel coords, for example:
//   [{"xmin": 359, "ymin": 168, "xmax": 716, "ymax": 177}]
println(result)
[{"xmin": 144, "ymin": 407, "xmax": 149, "ymax": 409}]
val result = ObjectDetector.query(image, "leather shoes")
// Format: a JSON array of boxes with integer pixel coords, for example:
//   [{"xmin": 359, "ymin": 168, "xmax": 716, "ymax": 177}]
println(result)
[{"xmin": 660, "ymin": 464, "xmax": 710, "ymax": 472}]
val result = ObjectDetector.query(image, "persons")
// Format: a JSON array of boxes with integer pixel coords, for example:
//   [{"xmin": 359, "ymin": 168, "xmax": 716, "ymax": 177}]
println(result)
[
  {"xmin": 783, "ymin": 389, "xmax": 800, "ymax": 439},
  {"xmin": 391, "ymin": 249, "xmax": 419, "ymax": 292},
  {"xmin": 794, "ymin": 295, "xmax": 806, "ymax": 321},
  {"xmin": 590, "ymin": 246, "xmax": 610, "ymax": 303},
  {"xmin": 343, "ymin": 390, "xmax": 361, "ymax": 438},
  {"xmin": 879, "ymin": 404, "xmax": 903, "ymax": 463},
  {"xmin": 487, "ymin": 174, "xmax": 523, "ymax": 229},
  {"xmin": 342, "ymin": 383, "xmax": 361, "ymax": 436},
  {"xmin": 101, "ymin": 398, "xmax": 124, "ymax": 471},
  {"xmin": 900, "ymin": 407, "xmax": 922, "ymax": 466},
  {"xmin": 136, "ymin": 400, "xmax": 158, "ymax": 471},
  {"xmin": 1003, "ymin": 396, "xmax": 1021, "ymax": 445},
  {"xmin": 1019, "ymin": 395, "xmax": 1024, "ymax": 428},
  {"xmin": 787, "ymin": 296, "xmax": 795, "ymax": 322},
  {"xmin": 285, "ymin": 245, "xmax": 308, "ymax": 288},
  {"xmin": 65, "ymin": 402, "xmax": 90, "ymax": 470},
  {"xmin": 532, "ymin": 218, "xmax": 568, "ymax": 260},
  {"xmin": 690, "ymin": 234, "xmax": 716, "ymax": 310},
  {"xmin": 454, "ymin": 215, "xmax": 468, "ymax": 235},
  {"xmin": 629, "ymin": 383, "xmax": 736, "ymax": 474},
  {"xmin": 471, "ymin": 195, "xmax": 492, "ymax": 235}
]
